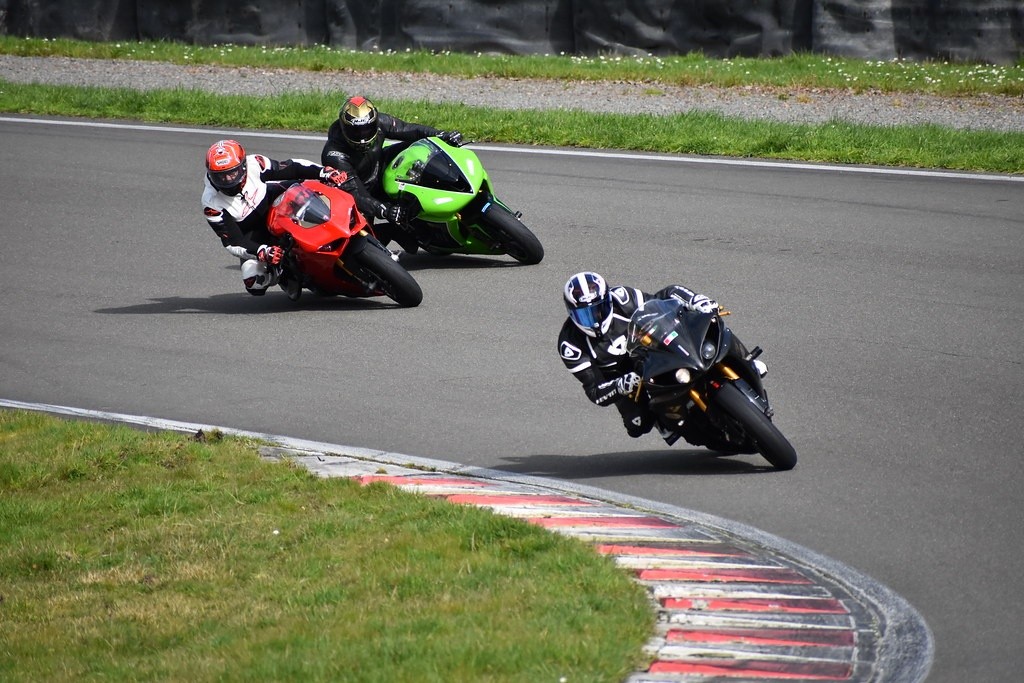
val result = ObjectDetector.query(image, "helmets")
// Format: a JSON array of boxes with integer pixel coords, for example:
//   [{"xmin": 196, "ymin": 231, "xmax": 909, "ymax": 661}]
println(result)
[
  {"xmin": 339, "ymin": 96, "xmax": 379, "ymax": 152},
  {"xmin": 564, "ymin": 272, "xmax": 614, "ymax": 337},
  {"xmin": 206, "ymin": 139, "xmax": 247, "ymax": 195}
]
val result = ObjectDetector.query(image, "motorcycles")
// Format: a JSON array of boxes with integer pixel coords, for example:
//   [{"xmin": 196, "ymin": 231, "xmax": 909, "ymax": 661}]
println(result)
[
  {"xmin": 622, "ymin": 298, "xmax": 798, "ymax": 469},
  {"xmin": 266, "ymin": 171, "xmax": 423, "ymax": 308},
  {"xmin": 371, "ymin": 135, "xmax": 546, "ymax": 264}
]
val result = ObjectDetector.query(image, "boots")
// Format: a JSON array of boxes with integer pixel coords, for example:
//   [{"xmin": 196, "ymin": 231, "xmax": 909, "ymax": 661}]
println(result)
[{"xmin": 377, "ymin": 224, "xmax": 417, "ymax": 252}]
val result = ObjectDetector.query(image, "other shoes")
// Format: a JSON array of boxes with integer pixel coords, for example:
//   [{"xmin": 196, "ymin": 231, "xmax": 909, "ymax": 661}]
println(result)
[
  {"xmin": 279, "ymin": 277, "xmax": 301, "ymax": 300},
  {"xmin": 755, "ymin": 359, "xmax": 767, "ymax": 378},
  {"xmin": 655, "ymin": 419, "xmax": 673, "ymax": 438}
]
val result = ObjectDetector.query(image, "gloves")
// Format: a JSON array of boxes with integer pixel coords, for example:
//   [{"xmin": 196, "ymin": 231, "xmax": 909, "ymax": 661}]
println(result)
[
  {"xmin": 618, "ymin": 371, "xmax": 640, "ymax": 395},
  {"xmin": 256, "ymin": 244, "xmax": 283, "ymax": 265},
  {"xmin": 320, "ymin": 166, "xmax": 348, "ymax": 187},
  {"xmin": 442, "ymin": 130, "xmax": 462, "ymax": 146},
  {"xmin": 688, "ymin": 294, "xmax": 719, "ymax": 314},
  {"xmin": 386, "ymin": 206, "xmax": 407, "ymax": 222}
]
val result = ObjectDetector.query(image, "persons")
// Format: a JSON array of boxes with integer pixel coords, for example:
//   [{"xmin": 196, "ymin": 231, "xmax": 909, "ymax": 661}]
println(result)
[
  {"xmin": 557, "ymin": 272, "xmax": 769, "ymax": 447},
  {"xmin": 203, "ymin": 140, "xmax": 358, "ymax": 295},
  {"xmin": 321, "ymin": 97, "xmax": 475, "ymax": 254}
]
